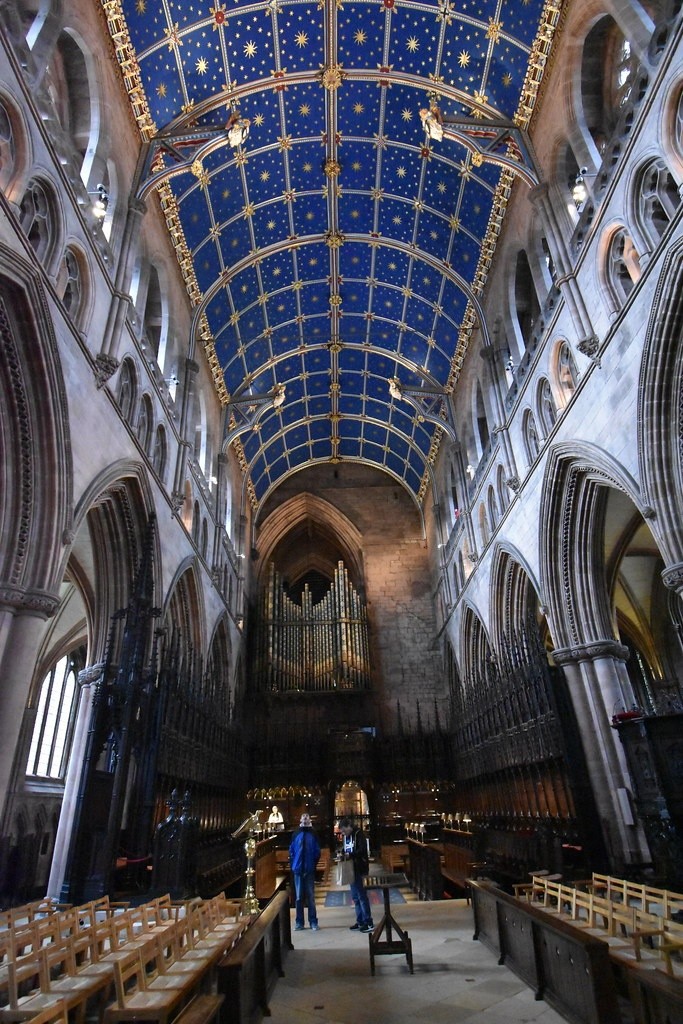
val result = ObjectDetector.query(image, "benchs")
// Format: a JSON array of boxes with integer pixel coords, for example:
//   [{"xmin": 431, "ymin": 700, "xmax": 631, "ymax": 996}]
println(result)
[
  {"xmin": 0, "ymin": 890, "xmax": 255, "ymax": 1023},
  {"xmin": 512, "ymin": 872, "xmax": 683, "ymax": 977}
]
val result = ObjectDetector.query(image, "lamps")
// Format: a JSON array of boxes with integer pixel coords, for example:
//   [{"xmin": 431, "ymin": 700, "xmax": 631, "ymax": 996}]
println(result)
[
  {"xmin": 447, "ymin": 814, "xmax": 455, "ymax": 830},
  {"xmin": 441, "ymin": 813, "xmax": 447, "ymax": 830},
  {"xmin": 404, "ymin": 823, "xmax": 419, "ymax": 842},
  {"xmin": 463, "ymin": 814, "xmax": 471, "ymax": 833},
  {"xmin": 419, "ymin": 824, "xmax": 427, "ymax": 844},
  {"xmin": 455, "ymin": 813, "xmax": 462, "ymax": 830}
]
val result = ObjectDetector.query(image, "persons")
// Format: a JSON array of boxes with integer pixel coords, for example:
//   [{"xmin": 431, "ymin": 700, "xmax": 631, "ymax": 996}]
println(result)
[
  {"xmin": 338, "ymin": 819, "xmax": 374, "ymax": 932},
  {"xmin": 290, "ymin": 813, "xmax": 320, "ymax": 930},
  {"xmin": 268, "ymin": 806, "xmax": 284, "ymax": 829}
]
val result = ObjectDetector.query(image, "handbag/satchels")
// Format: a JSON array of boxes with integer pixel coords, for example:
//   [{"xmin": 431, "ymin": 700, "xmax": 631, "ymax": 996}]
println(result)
[{"xmin": 336, "ymin": 855, "xmax": 356, "ymax": 886}]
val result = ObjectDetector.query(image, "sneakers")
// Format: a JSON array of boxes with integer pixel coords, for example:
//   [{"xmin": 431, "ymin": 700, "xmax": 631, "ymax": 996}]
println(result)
[
  {"xmin": 359, "ymin": 924, "xmax": 375, "ymax": 933},
  {"xmin": 293, "ymin": 925, "xmax": 304, "ymax": 931},
  {"xmin": 310, "ymin": 925, "xmax": 319, "ymax": 930},
  {"xmin": 349, "ymin": 923, "xmax": 363, "ymax": 931}
]
